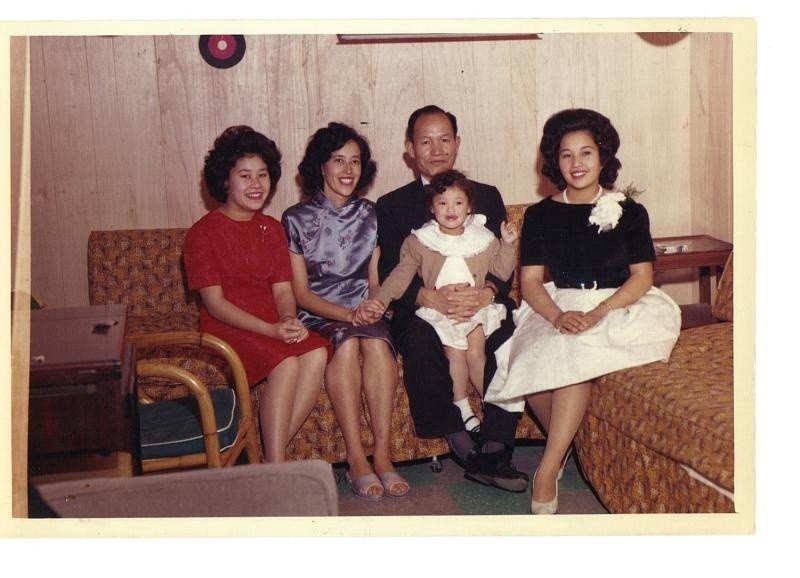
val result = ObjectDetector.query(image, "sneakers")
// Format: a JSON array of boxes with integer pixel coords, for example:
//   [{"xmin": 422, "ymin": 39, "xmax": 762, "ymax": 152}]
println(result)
[{"xmin": 464, "ymin": 448, "xmax": 529, "ymax": 491}]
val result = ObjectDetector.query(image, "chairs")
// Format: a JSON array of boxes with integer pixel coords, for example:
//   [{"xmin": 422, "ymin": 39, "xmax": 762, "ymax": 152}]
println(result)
[{"xmin": 128, "ymin": 332, "xmax": 261, "ymax": 472}]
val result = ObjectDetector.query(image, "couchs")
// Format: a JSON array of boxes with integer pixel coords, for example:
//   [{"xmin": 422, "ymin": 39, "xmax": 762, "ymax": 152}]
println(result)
[
  {"xmin": 573, "ymin": 248, "xmax": 734, "ymax": 513},
  {"xmin": 88, "ymin": 204, "xmax": 544, "ymax": 465}
]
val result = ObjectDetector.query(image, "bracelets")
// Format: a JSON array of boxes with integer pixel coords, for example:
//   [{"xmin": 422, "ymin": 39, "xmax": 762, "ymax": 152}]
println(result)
[
  {"xmin": 491, "ymin": 296, "xmax": 495, "ymax": 303},
  {"xmin": 603, "ymin": 301, "xmax": 612, "ymax": 312}
]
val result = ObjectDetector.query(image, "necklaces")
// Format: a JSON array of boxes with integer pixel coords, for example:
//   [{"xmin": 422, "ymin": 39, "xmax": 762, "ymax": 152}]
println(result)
[{"xmin": 562, "ymin": 184, "xmax": 602, "ymax": 204}]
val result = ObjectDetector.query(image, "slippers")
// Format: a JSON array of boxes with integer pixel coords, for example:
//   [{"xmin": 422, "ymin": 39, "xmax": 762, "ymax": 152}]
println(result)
[
  {"xmin": 381, "ymin": 472, "xmax": 412, "ymax": 496},
  {"xmin": 346, "ymin": 471, "xmax": 384, "ymax": 501}
]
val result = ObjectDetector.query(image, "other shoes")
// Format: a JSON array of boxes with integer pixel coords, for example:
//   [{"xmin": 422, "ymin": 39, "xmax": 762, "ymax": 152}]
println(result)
[
  {"xmin": 556, "ymin": 447, "xmax": 573, "ymax": 480},
  {"xmin": 531, "ymin": 471, "xmax": 558, "ymax": 514}
]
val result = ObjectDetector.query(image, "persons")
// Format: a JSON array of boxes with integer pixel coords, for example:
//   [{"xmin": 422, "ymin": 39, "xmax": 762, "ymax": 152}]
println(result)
[
  {"xmin": 363, "ymin": 168, "xmax": 520, "ymax": 446},
  {"xmin": 375, "ymin": 104, "xmax": 532, "ymax": 493},
  {"xmin": 482, "ymin": 108, "xmax": 681, "ymax": 514},
  {"xmin": 181, "ymin": 125, "xmax": 333, "ymax": 461},
  {"xmin": 280, "ymin": 122, "xmax": 412, "ymax": 503}
]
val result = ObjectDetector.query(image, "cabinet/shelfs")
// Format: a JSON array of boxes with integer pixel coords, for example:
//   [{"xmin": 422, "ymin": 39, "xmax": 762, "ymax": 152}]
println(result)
[
  {"xmin": 30, "ymin": 304, "xmax": 141, "ymax": 475},
  {"xmin": 652, "ymin": 234, "xmax": 733, "ymax": 328}
]
